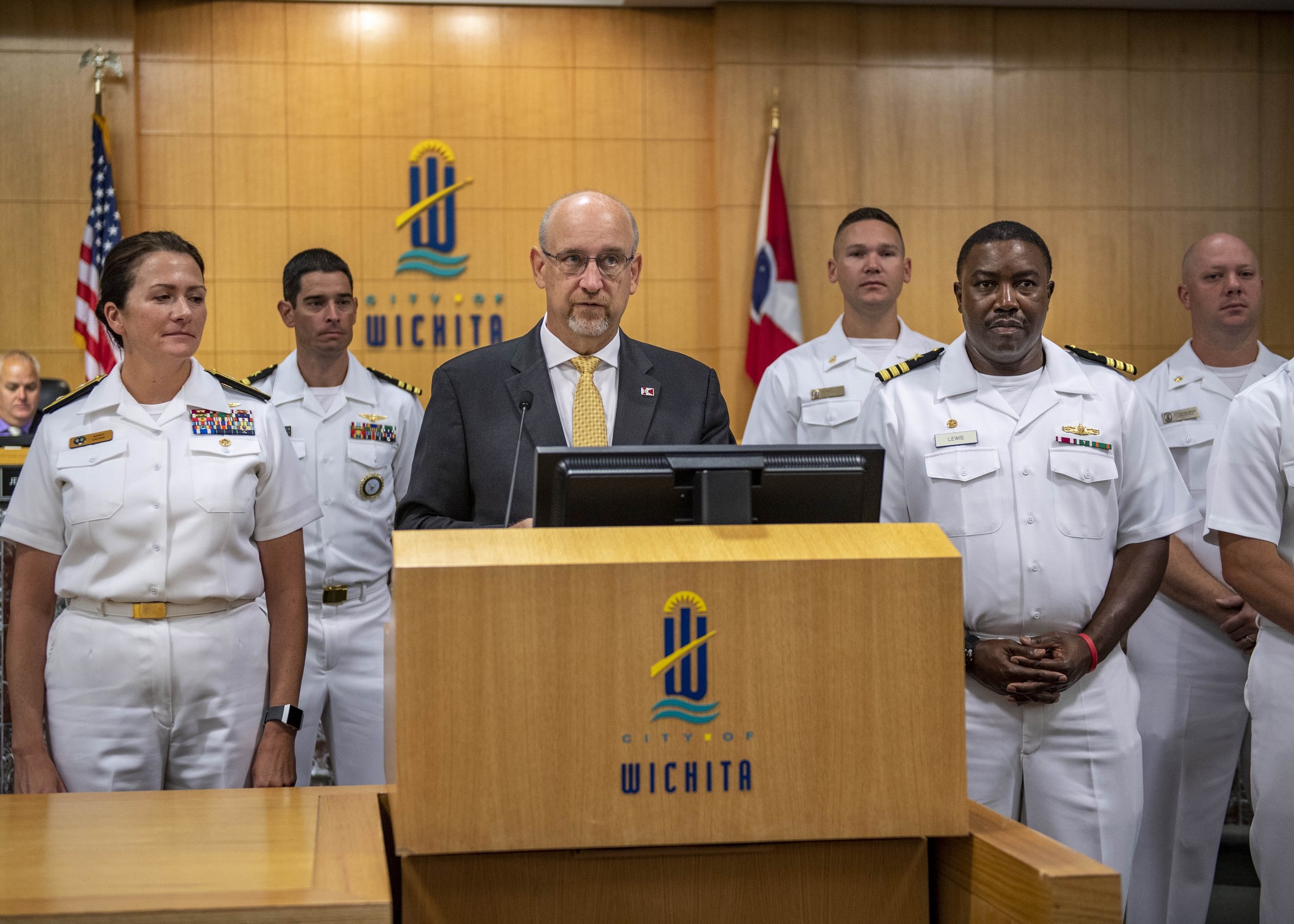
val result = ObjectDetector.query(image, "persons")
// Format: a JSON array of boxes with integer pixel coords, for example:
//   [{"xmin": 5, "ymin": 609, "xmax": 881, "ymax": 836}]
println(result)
[
  {"xmin": 234, "ymin": 247, "xmax": 425, "ymax": 786},
  {"xmin": 740, "ymin": 208, "xmax": 952, "ymax": 448},
  {"xmin": 0, "ymin": 229, "xmax": 325, "ymax": 796},
  {"xmin": 1203, "ymin": 357, "xmax": 1294, "ymax": 922},
  {"xmin": 1124, "ymin": 231, "xmax": 1288, "ymax": 923},
  {"xmin": 852, "ymin": 220, "xmax": 1199, "ymax": 923},
  {"xmin": 0, "ymin": 350, "xmax": 49, "ymax": 441},
  {"xmin": 393, "ymin": 187, "xmax": 740, "ymax": 527}
]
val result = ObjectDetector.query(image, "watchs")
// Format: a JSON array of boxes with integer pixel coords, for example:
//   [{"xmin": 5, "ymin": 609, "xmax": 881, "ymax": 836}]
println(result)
[
  {"xmin": 964, "ymin": 634, "xmax": 980, "ymax": 668},
  {"xmin": 264, "ymin": 704, "xmax": 305, "ymax": 732}
]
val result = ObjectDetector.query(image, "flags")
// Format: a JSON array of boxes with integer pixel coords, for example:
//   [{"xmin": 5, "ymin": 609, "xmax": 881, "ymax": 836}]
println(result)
[
  {"xmin": 73, "ymin": 135, "xmax": 126, "ymax": 383},
  {"xmin": 743, "ymin": 132, "xmax": 805, "ymax": 388}
]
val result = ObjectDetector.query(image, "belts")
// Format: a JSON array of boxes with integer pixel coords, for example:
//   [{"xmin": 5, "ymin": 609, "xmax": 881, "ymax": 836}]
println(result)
[
  {"xmin": 306, "ymin": 575, "xmax": 389, "ymax": 604},
  {"xmin": 69, "ymin": 595, "xmax": 253, "ymax": 621}
]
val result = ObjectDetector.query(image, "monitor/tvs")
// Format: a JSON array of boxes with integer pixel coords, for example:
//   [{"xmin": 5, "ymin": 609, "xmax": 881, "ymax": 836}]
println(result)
[{"xmin": 532, "ymin": 444, "xmax": 886, "ymax": 529}]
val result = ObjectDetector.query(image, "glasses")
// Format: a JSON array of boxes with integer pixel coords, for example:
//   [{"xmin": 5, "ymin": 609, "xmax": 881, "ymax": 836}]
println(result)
[{"xmin": 542, "ymin": 250, "xmax": 634, "ymax": 273}]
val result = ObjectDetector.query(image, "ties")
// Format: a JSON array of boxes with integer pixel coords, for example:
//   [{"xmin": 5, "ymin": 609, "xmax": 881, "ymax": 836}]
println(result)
[
  {"xmin": 9, "ymin": 426, "xmax": 21, "ymax": 436},
  {"xmin": 569, "ymin": 356, "xmax": 608, "ymax": 448}
]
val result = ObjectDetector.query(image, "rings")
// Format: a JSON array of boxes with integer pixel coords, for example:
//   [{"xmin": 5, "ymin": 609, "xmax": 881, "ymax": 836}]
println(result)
[{"xmin": 1245, "ymin": 636, "xmax": 1255, "ymax": 644}]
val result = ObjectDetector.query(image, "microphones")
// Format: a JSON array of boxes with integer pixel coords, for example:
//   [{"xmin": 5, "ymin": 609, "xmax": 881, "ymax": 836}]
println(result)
[{"xmin": 505, "ymin": 391, "xmax": 535, "ymax": 528}]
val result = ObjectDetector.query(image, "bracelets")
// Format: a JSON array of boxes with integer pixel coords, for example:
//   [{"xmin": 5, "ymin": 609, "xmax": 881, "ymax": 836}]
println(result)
[{"xmin": 1076, "ymin": 633, "xmax": 1098, "ymax": 673}]
